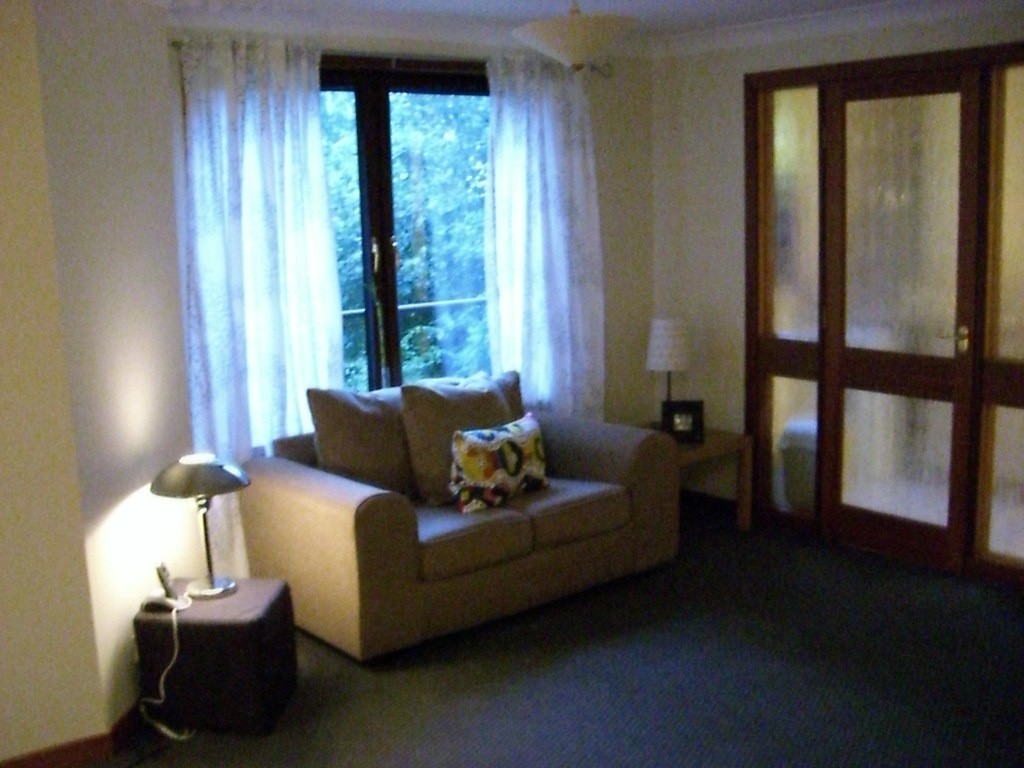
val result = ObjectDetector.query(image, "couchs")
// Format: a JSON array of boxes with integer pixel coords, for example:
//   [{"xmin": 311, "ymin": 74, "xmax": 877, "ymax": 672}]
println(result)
[{"xmin": 238, "ymin": 371, "xmax": 683, "ymax": 661}]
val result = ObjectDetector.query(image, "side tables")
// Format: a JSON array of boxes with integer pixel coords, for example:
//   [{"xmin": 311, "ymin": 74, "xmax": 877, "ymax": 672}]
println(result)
[
  {"xmin": 637, "ymin": 423, "xmax": 754, "ymax": 530},
  {"xmin": 133, "ymin": 577, "xmax": 298, "ymax": 736}
]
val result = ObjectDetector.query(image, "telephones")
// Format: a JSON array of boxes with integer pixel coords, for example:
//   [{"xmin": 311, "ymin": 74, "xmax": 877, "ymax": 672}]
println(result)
[{"xmin": 142, "ymin": 561, "xmax": 193, "ymax": 610}]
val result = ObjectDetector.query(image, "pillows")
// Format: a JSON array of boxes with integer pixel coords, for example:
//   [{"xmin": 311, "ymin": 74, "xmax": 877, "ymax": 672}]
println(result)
[
  {"xmin": 307, "ymin": 389, "xmax": 418, "ymax": 501},
  {"xmin": 400, "ymin": 370, "xmax": 551, "ymax": 508},
  {"xmin": 448, "ymin": 412, "xmax": 550, "ymax": 514}
]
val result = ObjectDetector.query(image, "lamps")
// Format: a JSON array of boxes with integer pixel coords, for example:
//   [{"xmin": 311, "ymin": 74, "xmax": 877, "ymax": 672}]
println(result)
[
  {"xmin": 150, "ymin": 453, "xmax": 252, "ymax": 601},
  {"xmin": 643, "ymin": 319, "xmax": 692, "ymax": 430}
]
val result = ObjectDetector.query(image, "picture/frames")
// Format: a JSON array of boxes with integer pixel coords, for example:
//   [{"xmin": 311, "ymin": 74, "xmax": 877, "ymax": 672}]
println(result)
[{"xmin": 662, "ymin": 400, "xmax": 704, "ymax": 443}]
